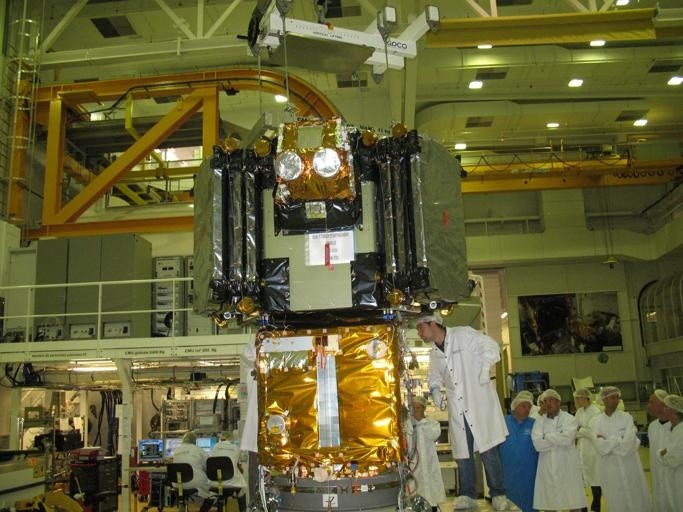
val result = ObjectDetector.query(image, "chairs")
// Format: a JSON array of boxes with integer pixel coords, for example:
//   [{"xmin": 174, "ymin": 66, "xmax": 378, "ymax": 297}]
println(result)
[
  {"xmin": 204, "ymin": 456, "xmax": 235, "ymax": 511},
  {"xmin": 166, "ymin": 463, "xmax": 197, "ymax": 512}
]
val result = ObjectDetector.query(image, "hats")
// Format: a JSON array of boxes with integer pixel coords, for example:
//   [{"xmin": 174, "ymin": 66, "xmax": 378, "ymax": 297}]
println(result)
[
  {"xmin": 411, "ymin": 396, "xmax": 427, "ymax": 406},
  {"xmin": 663, "ymin": 394, "xmax": 683, "ymax": 413},
  {"xmin": 654, "ymin": 387, "xmax": 669, "ymax": 402},
  {"xmin": 220, "ymin": 430, "xmax": 232, "ymax": 441},
  {"xmin": 541, "ymin": 388, "xmax": 561, "ymax": 401},
  {"xmin": 598, "ymin": 385, "xmax": 622, "ymax": 399},
  {"xmin": 183, "ymin": 431, "xmax": 197, "ymax": 443},
  {"xmin": 517, "ymin": 389, "xmax": 535, "ymax": 399},
  {"xmin": 573, "ymin": 387, "xmax": 592, "ymax": 399},
  {"xmin": 414, "ymin": 311, "xmax": 442, "ymax": 328},
  {"xmin": 510, "ymin": 395, "xmax": 533, "ymax": 411}
]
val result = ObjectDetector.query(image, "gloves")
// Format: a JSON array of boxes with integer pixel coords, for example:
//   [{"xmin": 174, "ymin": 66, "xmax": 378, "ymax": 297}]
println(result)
[{"xmin": 431, "ymin": 388, "xmax": 448, "ymax": 410}]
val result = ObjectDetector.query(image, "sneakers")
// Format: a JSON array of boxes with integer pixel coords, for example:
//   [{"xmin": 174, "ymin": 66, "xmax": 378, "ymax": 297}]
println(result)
[
  {"xmin": 452, "ymin": 496, "xmax": 478, "ymax": 510},
  {"xmin": 491, "ymin": 494, "xmax": 508, "ymax": 511}
]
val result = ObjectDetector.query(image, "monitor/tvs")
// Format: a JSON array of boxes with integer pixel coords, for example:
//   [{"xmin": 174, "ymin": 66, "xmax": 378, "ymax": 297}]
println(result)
[{"xmin": 137, "ymin": 439, "xmax": 164, "ymax": 463}]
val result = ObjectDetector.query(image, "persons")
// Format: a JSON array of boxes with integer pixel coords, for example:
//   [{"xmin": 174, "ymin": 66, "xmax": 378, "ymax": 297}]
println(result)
[
  {"xmin": 408, "ymin": 395, "xmax": 446, "ymax": 512},
  {"xmin": 198, "ymin": 432, "xmax": 246, "ymax": 512},
  {"xmin": 573, "ymin": 389, "xmax": 602, "ymax": 512},
  {"xmin": 173, "ymin": 433, "xmax": 210, "ymax": 500},
  {"xmin": 532, "ymin": 389, "xmax": 589, "ymax": 512},
  {"xmin": 658, "ymin": 395, "xmax": 683, "ymax": 512},
  {"xmin": 498, "ymin": 395, "xmax": 539, "ymax": 512},
  {"xmin": 648, "ymin": 390, "xmax": 669, "ymax": 512},
  {"xmin": 415, "ymin": 313, "xmax": 508, "ymax": 512},
  {"xmin": 589, "ymin": 386, "xmax": 650, "ymax": 511},
  {"xmin": 518, "ymin": 392, "xmax": 541, "ymax": 416}
]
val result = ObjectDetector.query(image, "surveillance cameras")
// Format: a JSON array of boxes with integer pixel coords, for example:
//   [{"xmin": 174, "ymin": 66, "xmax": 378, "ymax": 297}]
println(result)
[{"xmin": 608, "ymin": 256, "xmax": 620, "ymax": 268}]
